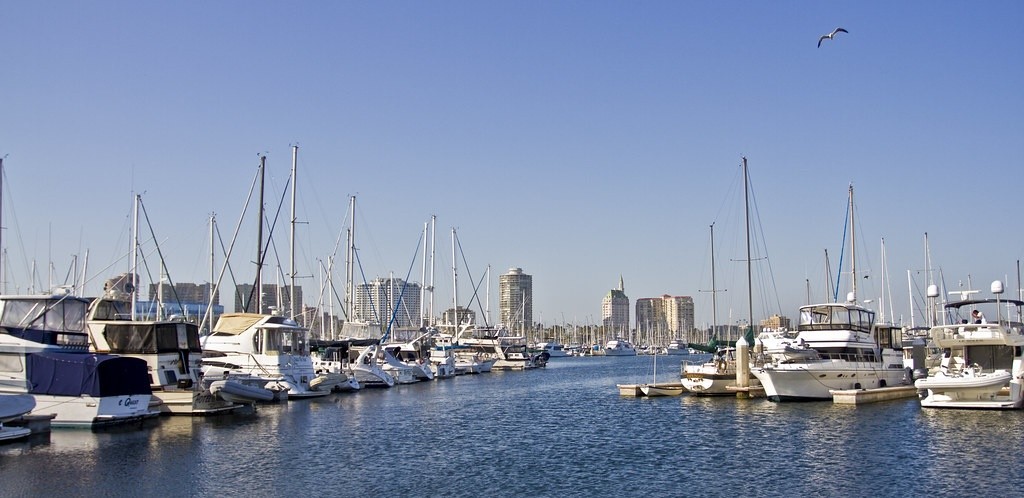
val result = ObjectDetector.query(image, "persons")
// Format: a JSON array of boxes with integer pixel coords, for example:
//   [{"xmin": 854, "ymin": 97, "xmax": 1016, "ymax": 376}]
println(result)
[
  {"xmin": 972, "ymin": 310, "xmax": 988, "ymax": 324},
  {"xmin": 961, "ymin": 319, "xmax": 968, "ymax": 324},
  {"xmin": 975, "ymin": 320, "xmax": 981, "ymax": 325}
]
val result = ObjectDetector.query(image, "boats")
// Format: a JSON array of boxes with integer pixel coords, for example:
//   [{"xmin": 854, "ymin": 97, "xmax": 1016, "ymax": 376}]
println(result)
[
  {"xmin": 664, "ymin": 332, "xmax": 689, "ymax": 355},
  {"xmin": 914, "ymin": 259, "xmax": 1024, "ymax": 410},
  {"xmin": 602, "ymin": 340, "xmax": 636, "ymax": 356},
  {"xmin": 536, "ymin": 343, "xmax": 567, "ymax": 357}
]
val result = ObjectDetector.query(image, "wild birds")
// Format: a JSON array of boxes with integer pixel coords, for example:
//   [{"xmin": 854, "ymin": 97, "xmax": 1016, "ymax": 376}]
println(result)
[{"xmin": 817, "ymin": 27, "xmax": 848, "ymax": 48}]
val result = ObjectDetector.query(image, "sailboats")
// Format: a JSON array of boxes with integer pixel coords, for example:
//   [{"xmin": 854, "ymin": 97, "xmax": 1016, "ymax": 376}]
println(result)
[
  {"xmin": 640, "ymin": 331, "xmax": 682, "ymax": 397},
  {"xmin": 0, "ymin": 144, "xmax": 550, "ymax": 430},
  {"xmin": 680, "ymin": 156, "xmax": 784, "ymax": 397},
  {"xmin": 750, "ymin": 181, "xmax": 907, "ymax": 403},
  {"xmin": 553, "ymin": 313, "xmax": 710, "ymax": 355}
]
